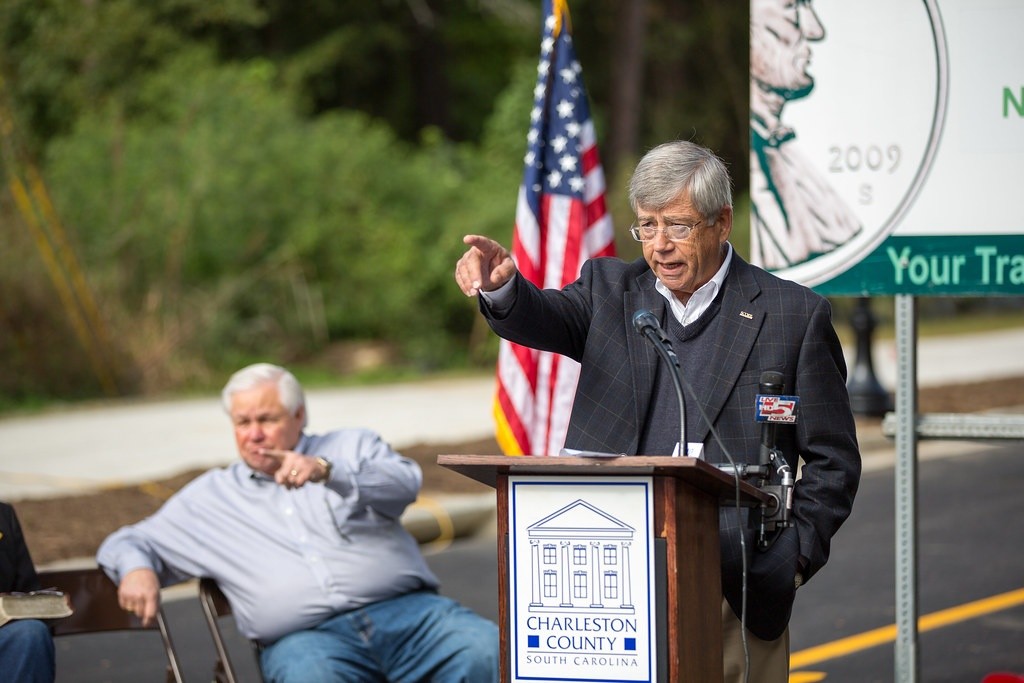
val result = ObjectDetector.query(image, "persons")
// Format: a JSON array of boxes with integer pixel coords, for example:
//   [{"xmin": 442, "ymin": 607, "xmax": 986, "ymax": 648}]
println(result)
[
  {"xmin": 0, "ymin": 501, "xmax": 59, "ymax": 683},
  {"xmin": 96, "ymin": 363, "xmax": 500, "ymax": 683},
  {"xmin": 457, "ymin": 140, "xmax": 862, "ymax": 682}
]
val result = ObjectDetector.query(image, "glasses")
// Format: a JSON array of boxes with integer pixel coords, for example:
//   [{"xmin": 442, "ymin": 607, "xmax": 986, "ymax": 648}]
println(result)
[{"xmin": 628, "ymin": 212, "xmax": 717, "ymax": 242}]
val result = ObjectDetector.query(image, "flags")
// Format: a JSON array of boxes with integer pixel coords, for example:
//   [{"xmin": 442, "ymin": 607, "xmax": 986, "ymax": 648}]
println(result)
[{"xmin": 493, "ymin": 1, "xmax": 615, "ymax": 457}]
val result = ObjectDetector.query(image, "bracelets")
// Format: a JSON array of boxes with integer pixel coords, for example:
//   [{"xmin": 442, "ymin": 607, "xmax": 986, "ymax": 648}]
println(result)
[{"xmin": 317, "ymin": 455, "xmax": 331, "ymax": 475}]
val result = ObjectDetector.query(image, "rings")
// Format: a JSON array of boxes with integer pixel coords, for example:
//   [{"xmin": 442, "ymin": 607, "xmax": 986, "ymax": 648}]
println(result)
[{"xmin": 291, "ymin": 470, "xmax": 297, "ymax": 475}]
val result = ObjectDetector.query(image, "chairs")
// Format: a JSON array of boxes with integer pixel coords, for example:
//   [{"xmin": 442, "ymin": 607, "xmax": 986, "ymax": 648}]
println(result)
[{"xmin": 37, "ymin": 567, "xmax": 238, "ymax": 683}]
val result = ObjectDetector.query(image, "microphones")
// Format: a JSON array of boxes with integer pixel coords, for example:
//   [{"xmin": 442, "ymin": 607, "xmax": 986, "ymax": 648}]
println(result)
[
  {"xmin": 755, "ymin": 370, "xmax": 786, "ymax": 489},
  {"xmin": 632, "ymin": 309, "xmax": 681, "ymax": 371}
]
